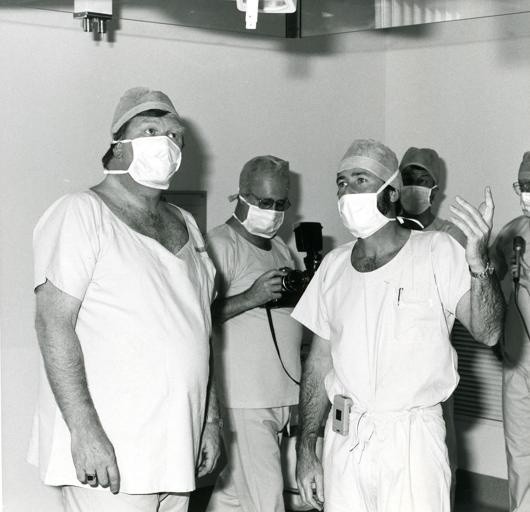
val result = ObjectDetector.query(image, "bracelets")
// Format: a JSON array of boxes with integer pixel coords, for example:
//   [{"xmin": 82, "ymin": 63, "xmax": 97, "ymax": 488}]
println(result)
[{"xmin": 205, "ymin": 416, "xmax": 221, "ymax": 423}]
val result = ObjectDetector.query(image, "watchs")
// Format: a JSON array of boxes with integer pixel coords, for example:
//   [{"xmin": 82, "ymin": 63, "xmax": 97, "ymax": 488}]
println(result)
[{"xmin": 469, "ymin": 259, "xmax": 496, "ymax": 279}]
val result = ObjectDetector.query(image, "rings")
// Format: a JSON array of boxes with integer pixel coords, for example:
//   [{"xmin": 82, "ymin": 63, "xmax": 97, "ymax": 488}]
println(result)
[{"xmin": 87, "ymin": 475, "xmax": 95, "ymax": 480}]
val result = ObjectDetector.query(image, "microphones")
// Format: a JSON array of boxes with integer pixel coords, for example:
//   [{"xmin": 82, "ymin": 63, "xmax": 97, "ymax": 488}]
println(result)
[{"xmin": 513, "ymin": 236, "xmax": 524, "ymax": 282}]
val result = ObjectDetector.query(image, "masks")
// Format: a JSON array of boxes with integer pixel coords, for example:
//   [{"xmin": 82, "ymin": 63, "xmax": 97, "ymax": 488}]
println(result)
[
  {"xmin": 112, "ymin": 140, "xmax": 181, "ymax": 189},
  {"xmin": 519, "ymin": 192, "xmax": 530, "ymax": 217},
  {"xmin": 338, "ymin": 177, "xmax": 399, "ymax": 238},
  {"xmin": 238, "ymin": 195, "xmax": 284, "ymax": 238},
  {"xmin": 400, "ymin": 184, "xmax": 440, "ymax": 214}
]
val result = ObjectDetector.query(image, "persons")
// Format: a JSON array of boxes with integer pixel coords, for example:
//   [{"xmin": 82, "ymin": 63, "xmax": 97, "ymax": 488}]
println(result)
[
  {"xmin": 26, "ymin": 86, "xmax": 222, "ymax": 512},
  {"xmin": 488, "ymin": 152, "xmax": 530, "ymax": 512},
  {"xmin": 205, "ymin": 155, "xmax": 303, "ymax": 512},
  {"xmin": 399, "ymin": 147, "xmax": 468, "ymax": 248},
  {"xmin": 289, "ymin": 139, "xmax": 506, "ymax": 512}
]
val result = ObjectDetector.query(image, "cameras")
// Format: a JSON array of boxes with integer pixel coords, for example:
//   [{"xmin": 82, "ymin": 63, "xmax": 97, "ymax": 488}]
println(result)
[{"xmin": 260, "ymin": 222, "xmax": 323, "ymax": 308}]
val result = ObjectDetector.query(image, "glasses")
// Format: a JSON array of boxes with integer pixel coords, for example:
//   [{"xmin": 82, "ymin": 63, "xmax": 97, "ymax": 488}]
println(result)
[
  {"xmin": 513, "ymin": 182, "xmax": 530, "ymax": 195},
  {"xmin": 250, "ymin": 192, "xmax": 291, "ymax": 211}
]
